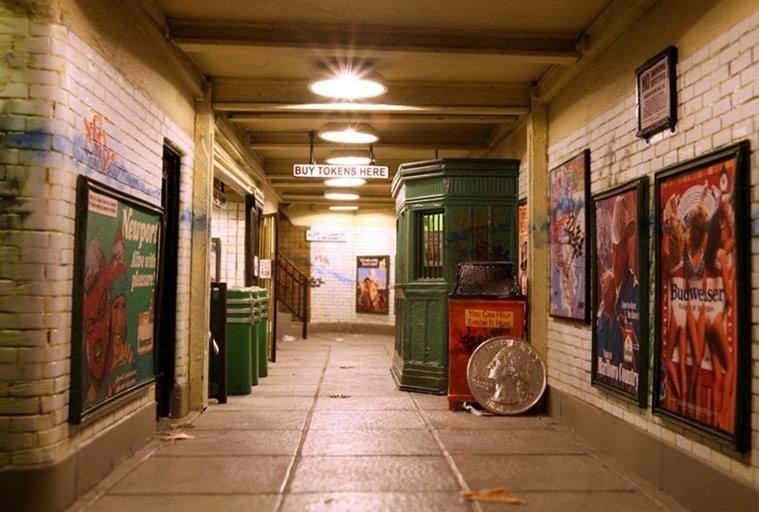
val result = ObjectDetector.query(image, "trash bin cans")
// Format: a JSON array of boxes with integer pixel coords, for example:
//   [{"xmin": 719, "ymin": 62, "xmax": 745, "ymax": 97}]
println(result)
[
  {"xmin": 447, "ymin": 260, "xmax": 527, "ymax": 411},
  {"xmin": 208, "ymin": 282, "xmax": 227, "ymax": 402}
]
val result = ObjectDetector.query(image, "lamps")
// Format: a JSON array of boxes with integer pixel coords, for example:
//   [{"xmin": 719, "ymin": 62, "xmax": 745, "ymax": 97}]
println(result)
[
  {"xmin": 317, "ymin": 121, "xmax": 379, "ymax": 145},
  {"xmin": 307, "ymin": 59, "xmax": 390, "ymax": 100},
  {"xmin": 324, "ymin": 149, "xmax": 373, "ymax": 166}
]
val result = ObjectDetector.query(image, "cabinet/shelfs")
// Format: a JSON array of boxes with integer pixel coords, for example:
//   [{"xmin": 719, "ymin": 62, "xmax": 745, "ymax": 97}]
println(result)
[{"xmin": 447, "ymin": 293, "xmax": 528, "ymax": 413}]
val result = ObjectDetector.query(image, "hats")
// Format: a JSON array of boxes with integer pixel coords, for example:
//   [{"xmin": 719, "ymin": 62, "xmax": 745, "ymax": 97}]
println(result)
[
  {"xmin": 363, "ymin": 276, "xmax": 372, "ymax": 283},
  {"xmin": 611, "ymin": 195, "xmax": 636, "ymax": 245}
]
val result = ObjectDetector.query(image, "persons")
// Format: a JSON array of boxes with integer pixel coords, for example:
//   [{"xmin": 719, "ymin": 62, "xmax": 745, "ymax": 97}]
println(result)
[
  {"xmin": 663, "ymin": 195, "xmax": 736, "ymax": 427},
  {"xmin": 83, "ymin": 238, "xmax": 137, "ymax": 408},
  {"xmin": 597, "ymin": 197, "xmax": 640, "ymax": 367},
  {"xmin": 358, "ymin": 278, "xmax": 379, "ymax": 309}
]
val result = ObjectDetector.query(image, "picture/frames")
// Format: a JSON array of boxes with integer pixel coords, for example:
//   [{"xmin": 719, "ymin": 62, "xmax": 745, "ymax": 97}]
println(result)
[
  {"xmin": 591, "ymin": 174, "xmax": 649, "ymax": 409},
  {"xmin": 354, "ymin": 254, "xmax": 390, "ymax": 315},
  {"xmin": 548, "ymin": 147, "xmax": 591, "ymax": 325},
  {"xmin": 69, "ymin": 173, "xmax": 167, "ymax": 426},
  {"xmin": 652, "ymin": 139, "xmax": 752, "ymax": 453}
]
